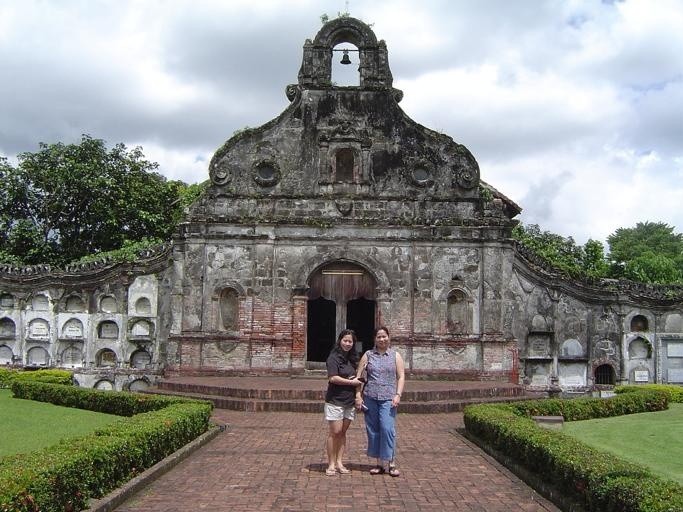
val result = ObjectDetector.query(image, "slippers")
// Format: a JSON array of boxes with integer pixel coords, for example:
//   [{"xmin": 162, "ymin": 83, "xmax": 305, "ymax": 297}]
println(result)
[
  {"xmin": 326, "ymin": 466, "xmax": 349, "ymax": 475},
  {"xmin": 389, "ymin": 467, "xmax": 400, "ymax": 476},
  {"xmin": 369, "ymin": 466, "xmax": 385, "ymax": 475}
]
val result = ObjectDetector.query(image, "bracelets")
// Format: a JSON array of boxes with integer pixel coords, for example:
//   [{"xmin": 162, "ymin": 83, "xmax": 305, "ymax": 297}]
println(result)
[{"xmin": 395, "ymin": 392, "xmax": 401, "ymax": 397}]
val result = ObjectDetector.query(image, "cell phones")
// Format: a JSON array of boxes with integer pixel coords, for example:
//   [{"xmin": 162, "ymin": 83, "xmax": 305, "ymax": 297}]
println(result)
[
  {"xmin": 358, "ymin": 378, "xmax": 365, "ymax": 382},
  {"xmin": 361, "ymin": 404, "xmax": 369, "ymax": 412}
]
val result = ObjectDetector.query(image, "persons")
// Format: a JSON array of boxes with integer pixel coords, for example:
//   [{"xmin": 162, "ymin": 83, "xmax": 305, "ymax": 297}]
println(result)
[
  {"xmin": 322, "ymin": 329, "xmax": 366, "ymax": 475},
  {"xmin": 353, "ymin": 326, "xmax": 405, "ymax": 476}
]
